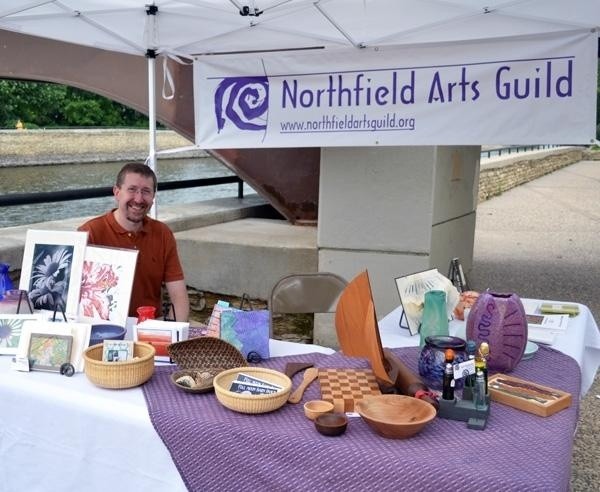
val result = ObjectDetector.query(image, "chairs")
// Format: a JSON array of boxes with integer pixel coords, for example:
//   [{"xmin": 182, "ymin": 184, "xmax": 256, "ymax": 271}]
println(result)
[{"xmin": 267, "ymin": 270, "xmax": 354, "ymax": 351}]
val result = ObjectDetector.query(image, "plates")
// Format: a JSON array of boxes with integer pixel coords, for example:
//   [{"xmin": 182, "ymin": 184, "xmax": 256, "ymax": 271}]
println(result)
[{"xmin": 521, "ymin": 340, "xmax": 538, "ymax": 360}]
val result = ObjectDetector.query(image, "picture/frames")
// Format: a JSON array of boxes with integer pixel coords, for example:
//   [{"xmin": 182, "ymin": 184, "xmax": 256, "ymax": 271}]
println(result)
[
  {"xmin": 450, "ymin": 254, "xmax": 469, "ymax": 291},
  {"xmin": 77, "ymin": 244, "xmax": 145, "ymax": 337},
  {"xmin": 0, "ymin": 313, "xmax": 49, "ymax": 356},
  {"xmin": 486, "ymin": 370, "xmax": 574, "ymax": 418},
  {"xmin": 102, "ymin": 338, "xmax": 135, "ymax": 364},
  {"xmin": 27, "ymin": 332, "xmax": 73, "ymax": 373},
  {"xmin": 18, "ymin": 228, "xmax": 89, "ymax": 317}
]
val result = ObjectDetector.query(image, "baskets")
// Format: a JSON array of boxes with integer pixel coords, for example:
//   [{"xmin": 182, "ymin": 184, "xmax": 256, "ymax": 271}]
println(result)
[
  {"xmin": 81, "ymin": 341, "xmax": 157, "ymax": 392},
  {"xmin": 210, "ymin": 366, "xmax": 292, "ymax": 416}
]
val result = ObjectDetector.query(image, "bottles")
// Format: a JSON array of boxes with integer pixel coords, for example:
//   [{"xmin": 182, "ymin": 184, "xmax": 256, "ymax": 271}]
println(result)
[
  {"xmin": 420, "ymin": 291, "xmax": 450, "ymax": 354},
  {"xmin": 136, "ymin": 306, "xmax": 156, "ymax": 325},
  {"xmin": 443, "ymin": 341, "xmax": 490, "ymax": 409}
]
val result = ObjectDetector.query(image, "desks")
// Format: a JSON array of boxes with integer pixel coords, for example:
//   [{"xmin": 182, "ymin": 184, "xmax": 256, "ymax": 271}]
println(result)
[{"xmin": 0, "ymin": 294, "xmax": 592, "ymax": 492}]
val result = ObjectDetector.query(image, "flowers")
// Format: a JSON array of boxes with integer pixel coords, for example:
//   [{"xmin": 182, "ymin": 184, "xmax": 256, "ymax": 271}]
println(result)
[
  {"xmin": 0, "ymin": 318, "xmax": 22, "ymax": 348},
  {"xmin": 26, "ymin": 245, "xmax": 73, "ymax": 308}
]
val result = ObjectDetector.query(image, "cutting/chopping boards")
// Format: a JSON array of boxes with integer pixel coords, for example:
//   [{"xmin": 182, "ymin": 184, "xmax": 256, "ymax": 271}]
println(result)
[{"xmin": 318, "ymin": 368, "xmax": 382, "ymax": 414}]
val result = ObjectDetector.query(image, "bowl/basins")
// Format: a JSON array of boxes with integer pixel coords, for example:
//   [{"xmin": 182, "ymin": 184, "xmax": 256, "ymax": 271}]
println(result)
[
  {"xmin": 304, "ymin": 400, "xmax": 335, "ymax": 421},
  {"xmin": 357, "ymin": 393, "xmax": 435, "ymax": 438},
  {"xmin": 314, "ymin": 414, "xmax": 347, "ymax": 437},
  {"xmin": 90, "ymin": 324, "xmax": 127, "ymax": 344}
]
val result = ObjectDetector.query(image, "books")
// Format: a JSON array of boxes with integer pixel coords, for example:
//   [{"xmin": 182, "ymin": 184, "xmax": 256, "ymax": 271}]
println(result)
[{"xmin": 525, "ymin": 304, "xmax": 579, "ymax": 346}]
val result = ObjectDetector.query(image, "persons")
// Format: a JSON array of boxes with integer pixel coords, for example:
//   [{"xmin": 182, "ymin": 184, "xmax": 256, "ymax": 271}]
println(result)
[{"xmin": 77, "ymin": 162, "xmax": 190, "ymax": 323}]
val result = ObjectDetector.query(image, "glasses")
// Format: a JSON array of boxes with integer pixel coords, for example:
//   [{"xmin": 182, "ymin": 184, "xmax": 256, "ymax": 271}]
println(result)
[{"xmin": 125, "ymin": 186, "xmax": 154, "ymax": 196}]
